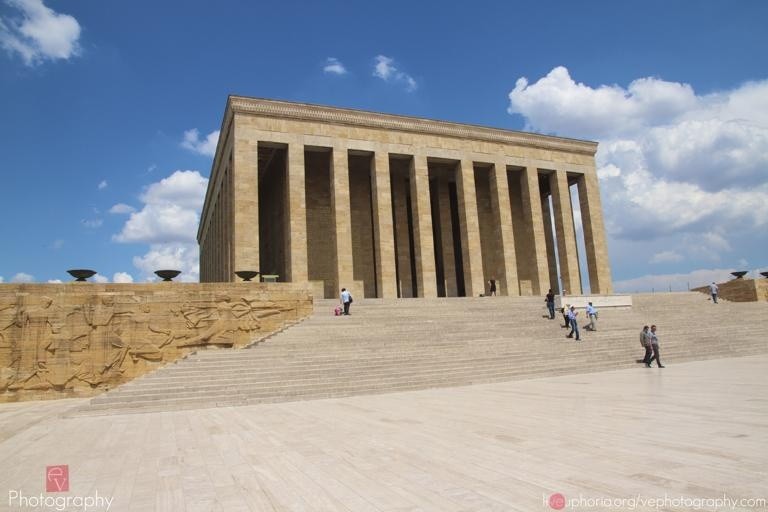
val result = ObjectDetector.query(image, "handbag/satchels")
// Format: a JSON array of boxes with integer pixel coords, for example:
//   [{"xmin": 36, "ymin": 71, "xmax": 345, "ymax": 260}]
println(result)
[{"xmin": 349, "ymin": 295, "xmax": 353, "ymax": 303}]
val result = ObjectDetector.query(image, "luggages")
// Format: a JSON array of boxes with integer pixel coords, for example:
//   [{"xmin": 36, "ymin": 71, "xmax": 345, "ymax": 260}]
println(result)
[{"xmin": 335, "ymin": 305, "xmax": 343, "ymax": 316}]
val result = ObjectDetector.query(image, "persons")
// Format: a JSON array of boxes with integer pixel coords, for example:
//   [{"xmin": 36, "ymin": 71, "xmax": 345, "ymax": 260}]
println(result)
[
  {"xmin": 640, "ymin": 325, "xmax": 665, "ymax": 368},
  {"xmin": 340, "ymin": 288, "xmax": 352, "ymax": 315},
  {"xmin": 586, "ymin": 302, "xmax": 598, "ymax": 331},
  {"xmin": 545, "ymin": 289, "xmax": 555, "ymax": 320},
  {"xmin": 709, "ymin": 281, "xmax": 719, "ymax": 304},
  {"xmin": 490, "ymin": 276, "xmax": 497, "ymax": 296},
  {"xmin": 562, "ymin": 303, "xmax": 581, "ymax": 340}
]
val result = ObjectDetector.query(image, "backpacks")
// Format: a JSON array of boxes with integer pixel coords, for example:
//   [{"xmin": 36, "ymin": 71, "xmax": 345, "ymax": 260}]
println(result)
[{"xmin": 561, "ymin": 308, "xmax": 564, "ymax": 314}]
